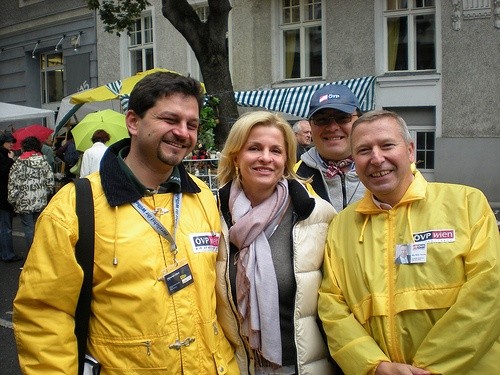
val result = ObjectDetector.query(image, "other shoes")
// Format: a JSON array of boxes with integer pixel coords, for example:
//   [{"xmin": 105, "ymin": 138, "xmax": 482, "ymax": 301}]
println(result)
[{"xmin": 4, "ymin": 255, "xmax": 22, "ymax": 263}]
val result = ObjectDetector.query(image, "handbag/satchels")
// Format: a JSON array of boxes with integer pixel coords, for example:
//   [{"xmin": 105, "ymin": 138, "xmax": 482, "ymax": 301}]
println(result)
[
  {"xmin": 77, "ymin": 354, "xmax": 100, "ymax": 375},
  {"xmin": 70, "ymin": 154, "xmax": 83, "ymax": 177}
]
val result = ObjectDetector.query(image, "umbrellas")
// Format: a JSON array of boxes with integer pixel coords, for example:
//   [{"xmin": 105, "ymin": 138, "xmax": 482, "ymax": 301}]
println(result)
[
  {"xmin": 11, "ymin": 125, "xmax": 54, "ymax": 151},
  {"xmin": 71, "ymin": 109, "xmax": 131, "ymax": 151}
]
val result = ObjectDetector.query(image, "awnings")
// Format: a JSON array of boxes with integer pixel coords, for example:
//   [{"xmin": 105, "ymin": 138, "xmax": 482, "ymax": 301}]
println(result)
[{"xmin": 203, "ymin": 74, "xmax": 379, "ymax": 118}]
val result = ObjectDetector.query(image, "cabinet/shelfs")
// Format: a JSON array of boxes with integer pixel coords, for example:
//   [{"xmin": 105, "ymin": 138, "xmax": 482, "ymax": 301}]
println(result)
[{"xmin": 182, "ymin": 158, "xmax": 219, "ymax": 198}]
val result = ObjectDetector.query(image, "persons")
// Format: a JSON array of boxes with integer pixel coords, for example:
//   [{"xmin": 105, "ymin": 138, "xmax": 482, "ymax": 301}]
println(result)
[
  {"xmin": 7, "ymin": 136, "xmax": 53, "ymax": 252},
  {"xmin": 292, "ymin": 120, "xmax": 312, "ymax": 163},
  {"xmin": 214, "ymin": 111, "xmax": 339, "ymax": 375},
  {"xmin": 80, "ymin": 129, "xmax": 110, "ymax": 178},
  {"xmin": 13, "ymin": 71, "xmax": 241, "ymax": 375},
  {"xmin": 395, "ymin": 244, "xmax": 410, "ymax": 263},
  {"xmin": 318, "ymin": 110, "xmax": 500, "ymax": 375},
  {"xmin": 192, "ymin": 143, "xmax": 211, "ymax": 168},
  {"xmin": 41, "ymin": 135, "xmax": 55, "ymax": 170},
  {"xmin": 292, "ymin": 84, "xmax": 367, "ymax": 213},
  {"xmin": 0, "ymin": 132, "xmax": 25, "ymax": 263},
  {"xmin": 55, "ymin": 128, "xmax": 81, "ymax": 185}
]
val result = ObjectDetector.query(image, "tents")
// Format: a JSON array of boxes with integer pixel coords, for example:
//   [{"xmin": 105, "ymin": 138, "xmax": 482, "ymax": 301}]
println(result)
[
  {"xmin": 52, "ymin": 67, "xmax": 207, "ymax": 175},
  {"xmin": 0, "ymin": 102, "xmax": 55, "ymax": 129}
]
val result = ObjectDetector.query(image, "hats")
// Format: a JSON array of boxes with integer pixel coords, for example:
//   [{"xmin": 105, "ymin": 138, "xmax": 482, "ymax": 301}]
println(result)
[
  {"xmin": 306, "ymin": 84, "xmax": 361, "ymax": 121},
  {"xmin": 0, "ymin": 133, "xmax": 16, "ymax": 146}
]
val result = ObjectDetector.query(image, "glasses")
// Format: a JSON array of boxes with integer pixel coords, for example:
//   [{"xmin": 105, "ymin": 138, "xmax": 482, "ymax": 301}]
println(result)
[{"xmin": 310, "ymin": 114, "xmax": 359, "ymax": 127}]
